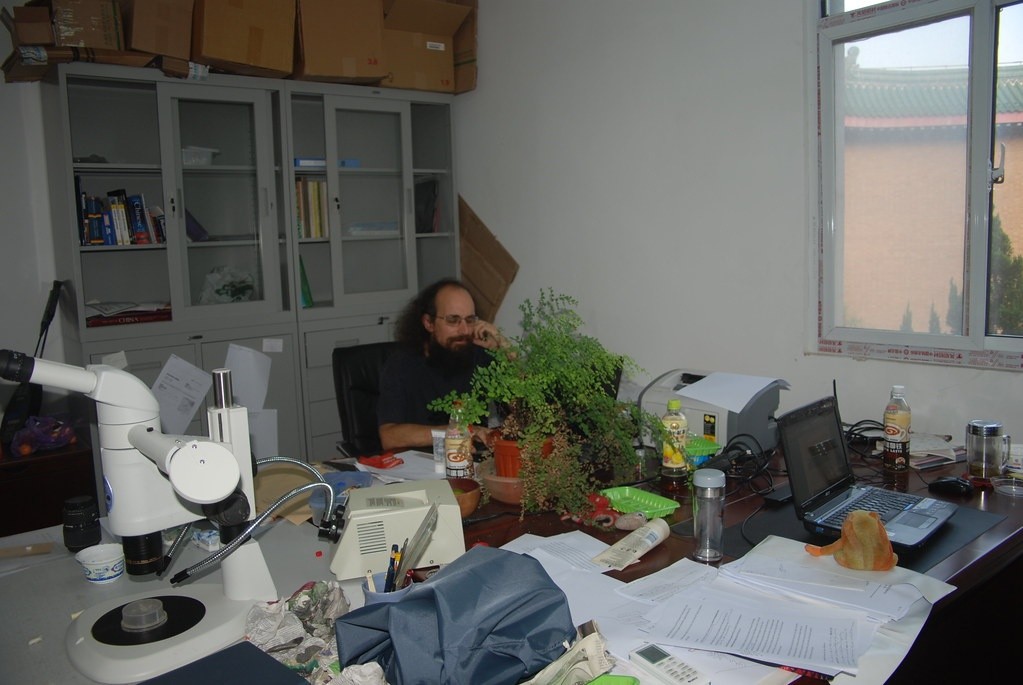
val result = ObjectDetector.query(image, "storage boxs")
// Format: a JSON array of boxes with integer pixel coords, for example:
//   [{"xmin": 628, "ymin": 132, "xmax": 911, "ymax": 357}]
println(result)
[{"xmin": 0, "ymin": 0, "xmax": 471, "ymax": 93}]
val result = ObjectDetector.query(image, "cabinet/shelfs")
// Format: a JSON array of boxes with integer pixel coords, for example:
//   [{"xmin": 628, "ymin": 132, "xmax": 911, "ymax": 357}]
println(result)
[{"xmin": 56, "ymin": 59, "xmax": 462, "ymax": 467}]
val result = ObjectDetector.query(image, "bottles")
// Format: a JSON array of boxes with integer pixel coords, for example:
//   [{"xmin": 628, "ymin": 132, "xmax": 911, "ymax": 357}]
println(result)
[
  {"xmin": 692, "ymin": 468, "xmax": 726, "ymax": 562},
  {"xmin": 883, "ymin": 384, "xmax": 912, "ymax": 471},
  {"xmin": 62, "ymin": 496, "xmax": 102, "ymax": 553},
  {"xmin": 659, "ymin": 398, "xmax": 689, "ymax": 486},
  {"xmin": 445, "ymin": 400, "xmax": 473, "ymax": 480}
]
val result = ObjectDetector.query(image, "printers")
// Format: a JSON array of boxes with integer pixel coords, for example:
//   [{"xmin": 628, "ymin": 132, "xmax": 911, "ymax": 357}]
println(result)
[{"xmin": 635, "ymin": 368, "xmax": 781, "ymax": 457}]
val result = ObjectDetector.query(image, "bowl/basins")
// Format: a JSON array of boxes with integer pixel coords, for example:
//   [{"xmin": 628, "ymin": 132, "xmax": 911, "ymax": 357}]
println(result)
[
  {"xmin": 75, "ymin": 542, "xmax": 125, "ymax": 586},
  {"xmin": 442, "ymin": 478, "xmax": 481, "ymax": 518},
  {"xmin": 182, "ymin": 144, "xmax": 219, "ymax": 167}
]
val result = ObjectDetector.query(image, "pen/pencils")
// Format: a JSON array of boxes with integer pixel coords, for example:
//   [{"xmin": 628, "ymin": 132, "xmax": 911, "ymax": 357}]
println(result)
[{"xmin": 366, "ymin": 543, "xmax": 414, "ymax": 592}]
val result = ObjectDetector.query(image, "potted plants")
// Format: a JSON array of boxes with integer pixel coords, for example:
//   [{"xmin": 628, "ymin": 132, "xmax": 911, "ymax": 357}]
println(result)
[{"xmin": 425, "ymin": 291, "xmax": 696, "ymax": 535}]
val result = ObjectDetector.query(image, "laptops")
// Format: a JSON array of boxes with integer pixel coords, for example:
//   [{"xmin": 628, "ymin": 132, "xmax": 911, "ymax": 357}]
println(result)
[{"xmin": 776, "ymin": 396, "xmax": 960, "ymax": 548}]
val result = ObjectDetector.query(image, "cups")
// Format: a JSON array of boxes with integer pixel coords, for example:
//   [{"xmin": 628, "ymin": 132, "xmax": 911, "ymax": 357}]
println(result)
[
  {"xmin": 430, "ymin": 429, "xmax": 444, "ymax": 475},
  {"xmin": 966, "ymin": 419, "xmax": 1011, "ymax": 480},
  {"xmin": 362, "ymin": 571, "xmax": 415, "ymax": 608}
]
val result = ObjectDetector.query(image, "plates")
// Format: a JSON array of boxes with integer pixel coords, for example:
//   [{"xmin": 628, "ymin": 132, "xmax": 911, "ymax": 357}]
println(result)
[
  {"xmin": 599, "ymin": 486, "xmax": 681, "ymax": 519},
  {"xmin": 989, "ymin": 475, "xmax": 1023, "ymax": 498}
]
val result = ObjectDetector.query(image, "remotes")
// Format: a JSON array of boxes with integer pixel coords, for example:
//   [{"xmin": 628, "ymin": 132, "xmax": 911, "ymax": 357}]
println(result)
[{"xmin": 628, "ymin": 643, "xmax": 707, "ymax": 685}]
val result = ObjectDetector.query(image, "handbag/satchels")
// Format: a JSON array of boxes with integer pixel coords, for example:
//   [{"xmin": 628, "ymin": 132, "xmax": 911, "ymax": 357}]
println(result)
[{"xmin": 333, "ymin": 544, "xmax": 577, "ymax": 685}]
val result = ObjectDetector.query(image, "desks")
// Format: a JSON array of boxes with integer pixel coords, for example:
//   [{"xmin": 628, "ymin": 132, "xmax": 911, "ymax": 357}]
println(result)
[{"xmin": 0, "ymin": 446, "xmax": 1023, "ymax": 685}]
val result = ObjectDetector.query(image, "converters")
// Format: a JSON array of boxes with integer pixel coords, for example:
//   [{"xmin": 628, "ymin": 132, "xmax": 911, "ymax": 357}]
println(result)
[{"xmin": 764, "ymin": 486, "xmax": 793, "ymax": 508}]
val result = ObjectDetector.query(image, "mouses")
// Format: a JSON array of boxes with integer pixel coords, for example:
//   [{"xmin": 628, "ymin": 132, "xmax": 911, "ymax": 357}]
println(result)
[{"xmin": 929, "ymin": 476, "xmax": 973, "ymax": 496}]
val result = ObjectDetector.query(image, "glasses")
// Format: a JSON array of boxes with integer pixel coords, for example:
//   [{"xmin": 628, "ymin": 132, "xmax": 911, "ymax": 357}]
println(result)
[{"xmin": 428, "ymin": 313, "xmax": 479, "ymax": 328}]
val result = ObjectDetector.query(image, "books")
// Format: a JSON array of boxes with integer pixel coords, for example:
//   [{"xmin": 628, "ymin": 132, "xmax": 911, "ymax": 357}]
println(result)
[
  {"xmin": 185, "ymin": 208, "xmax": 211, "ymax": 243},
  {"xmin": 296, "ymin": 176, "xmax": 329, "ymax": 237},
  {"xmin": 873, "ymin": 432, "xmax": 969, "ymax": 470},
  {"xmin": 415, "ymin": 166, "xmax": 443, "ymax": 233},
  {"xmin": 76, "ymin": 175, "xmax": 166, "ymax": 245}
]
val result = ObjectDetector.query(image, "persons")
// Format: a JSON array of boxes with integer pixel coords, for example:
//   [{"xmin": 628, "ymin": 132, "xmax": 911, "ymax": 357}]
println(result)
[{"xmin": 378, "ymin": 275, "xmax": 518, "ymax": 450}]
